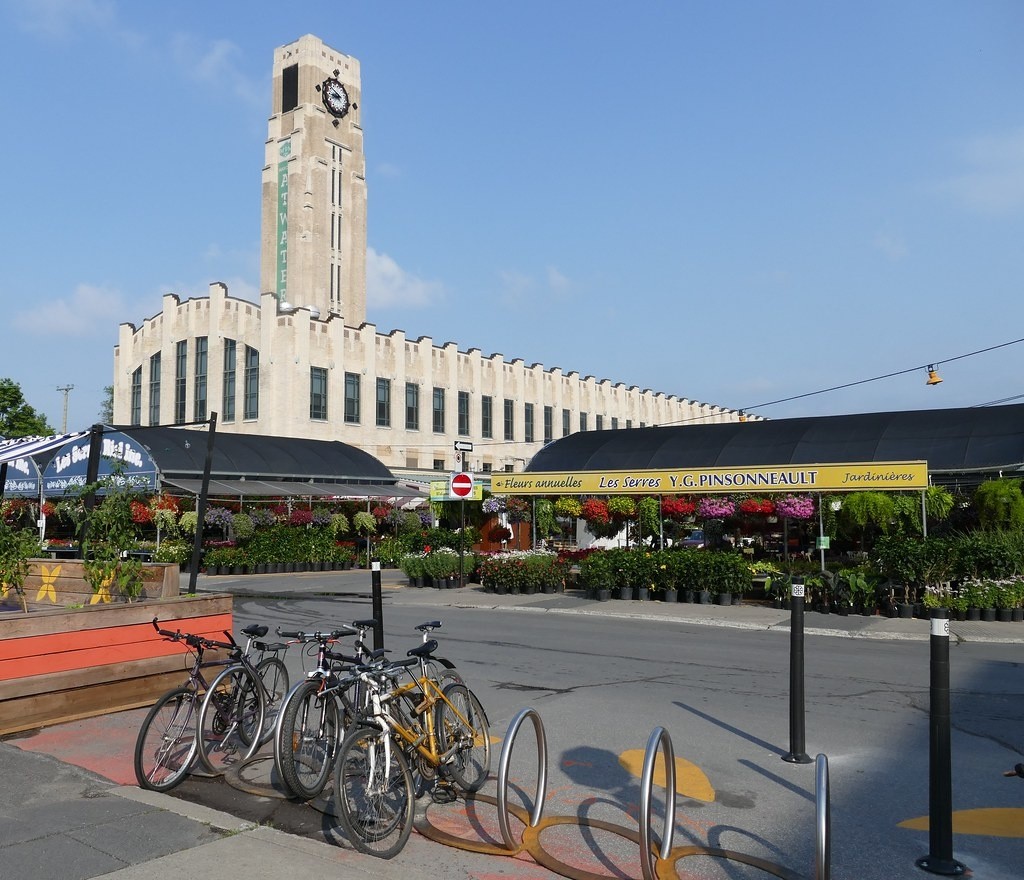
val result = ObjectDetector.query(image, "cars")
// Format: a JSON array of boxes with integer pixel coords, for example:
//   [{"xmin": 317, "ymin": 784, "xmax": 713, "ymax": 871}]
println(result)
[{"xmin": 670, "ymin": 531, "xmax": 729, "ymax": 552}]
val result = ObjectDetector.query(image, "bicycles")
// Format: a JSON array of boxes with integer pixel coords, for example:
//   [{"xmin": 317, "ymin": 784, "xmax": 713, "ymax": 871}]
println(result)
[{"xmin": 134, "ymin": 618, "xmax": 488, "ymax": 859}]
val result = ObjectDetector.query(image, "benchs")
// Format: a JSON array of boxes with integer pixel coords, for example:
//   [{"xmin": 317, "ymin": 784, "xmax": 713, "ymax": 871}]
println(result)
[
  {"xmin": 42, "ymin": 549, "xmax": 100, "ymax": 560},
  {"xmin": 126, "ymin": 551, "xmax": 157, "ymax": 563}
]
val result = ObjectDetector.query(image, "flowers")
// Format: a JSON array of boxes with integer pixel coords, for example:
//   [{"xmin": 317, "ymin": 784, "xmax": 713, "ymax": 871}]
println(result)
[
  {"xmin": 420, "ymin": 545, "xmax": 432, "ymax": 555},
  {"xmin": 128, "ymin": 482, "xmax": 432, "ymax": 524},
  {"xmin": 477, "ymin": 559, "xmax": 573, "ymax": 581},
  {"xmin": 4, "ymin": 492, "xmax": 61, "ymax": 526},
  {"xmin": 418, "ymin": 530, "xmax": 430, "ymax": 537},
  {"xmin": 481, "ymin": 494, "xmax": 814, "ymax": 521}
]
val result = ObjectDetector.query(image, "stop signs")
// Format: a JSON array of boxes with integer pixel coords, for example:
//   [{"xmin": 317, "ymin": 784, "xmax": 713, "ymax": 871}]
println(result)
[{"xmin": 449, "ymin": 472, "xmax": 474, "ymax": 498}]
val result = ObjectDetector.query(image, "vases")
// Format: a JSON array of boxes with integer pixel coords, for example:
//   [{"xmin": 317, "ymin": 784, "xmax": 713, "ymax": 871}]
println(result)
[{"xmin": 483, "ymin": 582, "xmax": 567, "ymax": 595}]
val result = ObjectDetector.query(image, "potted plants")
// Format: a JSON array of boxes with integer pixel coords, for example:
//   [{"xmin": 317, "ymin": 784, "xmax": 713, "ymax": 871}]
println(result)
[
  {"xmin": 197, "ymin": 526, "xmax": 352, "ymax": 576},
  {"xmin": 359, "ymin": 526, "xmax": 473, "ymax": 590},
  {"xmin": 772, "ymin": 525, "xmax": 1024, "ymax": 624},
  {"xmin": 590, "ymin": 550, "xmax": 756, "ymax": 606}
]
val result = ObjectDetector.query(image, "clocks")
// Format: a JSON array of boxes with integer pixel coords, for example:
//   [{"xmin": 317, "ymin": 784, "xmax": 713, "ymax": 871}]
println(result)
[{"xmin": 322, "ymin": 77, "xmax": 352, "ymax": 120}]
